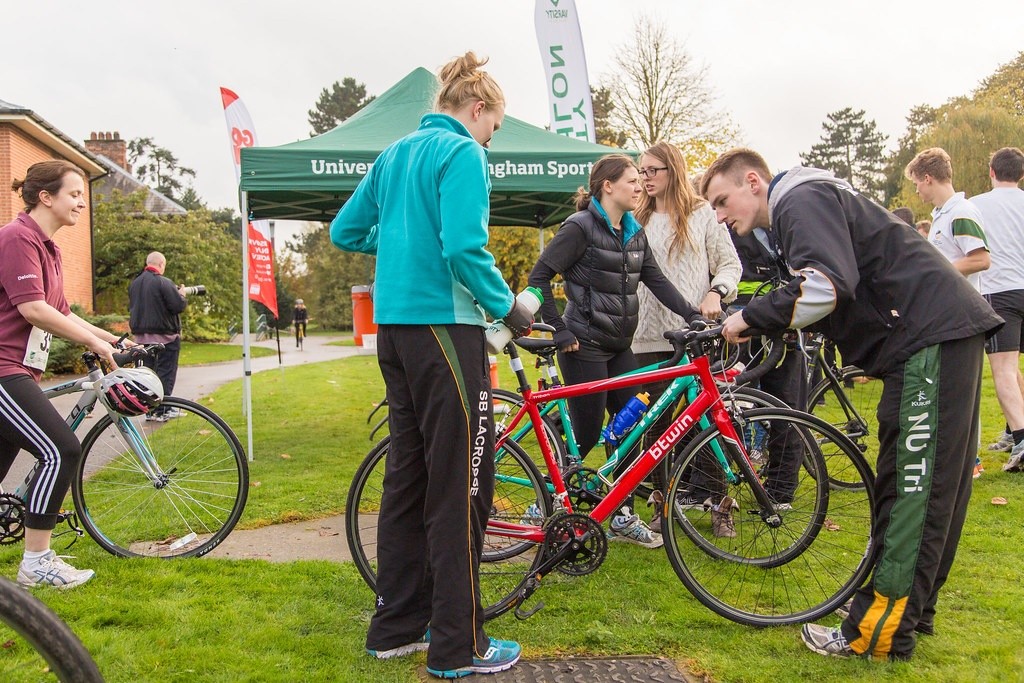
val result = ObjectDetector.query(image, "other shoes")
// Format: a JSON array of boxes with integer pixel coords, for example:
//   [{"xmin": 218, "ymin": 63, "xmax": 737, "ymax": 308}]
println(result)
[
  {"xmin": 646, "ymin": 489, "xmax": 667, "ymax": 533},
  {"xmin": 988, "ymin": 432, "xmax": 1016, "ymax": 451},
  {"xmin": 676, "ymin": 493, "xmax": 710, "ymax": 512},
  {"xmin": 704, "ymin": 496, "xmax": 740, "ymax": 537},
  {"xmin": 165, "ymin": 408, "xmax": 188, "ymax": 417},
  {"xmin": 518, "ymin": 503, "xmax": 545, "ymax": 527},
  {"xmin": 145, "ymin": 412, "xmax": 169, "ymax": 422},
  {"xmin": 750, "ymin": 449, "xmax": 763, "ymax": 460},
  {"xmin": 771, "ymin": 501, "xmax": 793, "ymax": 511},
  {"xmin": 972, "ymin": 463, "xmax": 985, "ymax": 478},
  {"xmin": 816, "ymin": 400, "xmax": 826, "ymax": 406}
]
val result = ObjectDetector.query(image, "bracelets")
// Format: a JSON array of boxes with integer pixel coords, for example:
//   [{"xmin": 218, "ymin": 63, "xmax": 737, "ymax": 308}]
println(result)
[{"xmin": 707, "ymin": 290, "xmax": 722, "ymax": 299}]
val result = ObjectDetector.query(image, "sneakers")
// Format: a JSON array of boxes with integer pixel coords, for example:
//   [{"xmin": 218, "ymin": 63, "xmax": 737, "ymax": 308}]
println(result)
[
  {"xmin": 364, "ymin": 628, "xmax": 431, "ymax": 658},
  {"xmin": 834, "ymin": 596, "xmax": 855, "ymax": 619},
  {"xmin": 1001, "ymin": 439, "xmax": 1024, "ymax": 471},
  {"xmin": 426, "ymin": 637, "xmax": 521, "ymax": 678},
  {"xmin": 17, "ymin": 549, "xmax": 95, "ymax": 591},
  {"xmin": 801, "ymin": 622, "xmax": 890, "ymax": 666},
  {"xmin": 605, "ymin": 512, "xmax": 664, "ymax": 549}
]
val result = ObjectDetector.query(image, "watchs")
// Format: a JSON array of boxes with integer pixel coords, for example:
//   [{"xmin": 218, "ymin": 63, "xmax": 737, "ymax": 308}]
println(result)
[{"xmin": 711, "ymin": 284, "xmax": 728, "ymax": 298}]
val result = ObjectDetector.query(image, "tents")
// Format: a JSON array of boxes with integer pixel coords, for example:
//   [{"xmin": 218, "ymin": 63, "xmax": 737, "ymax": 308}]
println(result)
[{"xmin": 241, "ymin": 67, "xmax": 645, "ymax": 462}]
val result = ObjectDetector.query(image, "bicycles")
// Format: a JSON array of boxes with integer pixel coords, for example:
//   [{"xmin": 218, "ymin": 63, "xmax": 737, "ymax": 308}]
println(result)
[
  {"xmin": 294, "ymin": 320, "xmax": 306, "ymax": 351},
  {"xmin": 343, "ymin": 276, "xmax": 889, "ymax": 626},
  {"xmin": 0, "ymin": 331, "xmax": 248, "ymax": 683}
]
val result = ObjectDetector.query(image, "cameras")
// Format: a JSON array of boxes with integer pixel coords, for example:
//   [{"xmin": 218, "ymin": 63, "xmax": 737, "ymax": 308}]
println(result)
[{"xmin": 177, "ymin": 285, "xmax": 206, "ymax": 295}]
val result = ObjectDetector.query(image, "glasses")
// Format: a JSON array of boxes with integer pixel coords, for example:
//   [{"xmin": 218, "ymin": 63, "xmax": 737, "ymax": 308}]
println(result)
[{"xmin": 638, "ymin": 167, "xmax": 668, "ymax": 178}]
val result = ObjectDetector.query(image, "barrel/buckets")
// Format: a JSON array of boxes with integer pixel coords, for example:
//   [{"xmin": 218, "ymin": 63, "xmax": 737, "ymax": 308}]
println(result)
[{"xmin": 352, "ymin": 285, "xmax": 377, "ymax": 345}]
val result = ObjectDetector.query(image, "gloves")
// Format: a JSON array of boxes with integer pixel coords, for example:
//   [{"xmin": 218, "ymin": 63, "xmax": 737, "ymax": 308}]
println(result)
[{"xmin": 504, "ymin": 296, "xmax": 537, "ymax": 339}]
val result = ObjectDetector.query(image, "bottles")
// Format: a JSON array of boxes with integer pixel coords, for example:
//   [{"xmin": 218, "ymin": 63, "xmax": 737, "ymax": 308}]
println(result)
[
  {"xmin": 603, "ymin": 391, "xmax": 651, "ymax": 447},
  {"xmin": 488, "ymin": 355, "xmax": 499, "ymax": 404},
  {"xmin": 485, "ymin": 286, "xmax": 545, "ymax": 354}
]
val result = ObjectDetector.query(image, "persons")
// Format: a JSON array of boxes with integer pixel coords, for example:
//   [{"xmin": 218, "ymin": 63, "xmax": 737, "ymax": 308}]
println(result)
[
  {"xmin": 702, "ymin": 150, "xmax": 1007, "ymax": 660},
  {"xmin": 0, "ymin": 160, "xmax": 145, "ymax": 592},
  {"xmin": 891, "ymin": 145, "xmax": 1024, "ymax": 477},
  {"xmin": 330, "ymin": 52, "xmax": 534, "ymax": 678},
  {"xmin": 129, "ymin": 252, "xmax": 188, "ymax": 422},
  {"xmin": 528, "ymin": 142, "xmax": 855, "ymax": 548},
  {"xmin": 293, "ymin": 299, "xmax": 309, "ymax": 347}
]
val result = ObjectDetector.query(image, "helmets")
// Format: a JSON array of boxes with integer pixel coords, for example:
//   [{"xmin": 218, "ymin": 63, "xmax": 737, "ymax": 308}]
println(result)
[
  {"xmin": 295, "ymin": 299, "xmax": 304, "ymax": 304},
  {"xmin": 93, "ymin": 365, "xmax": 165, "ymax": 417}
]
